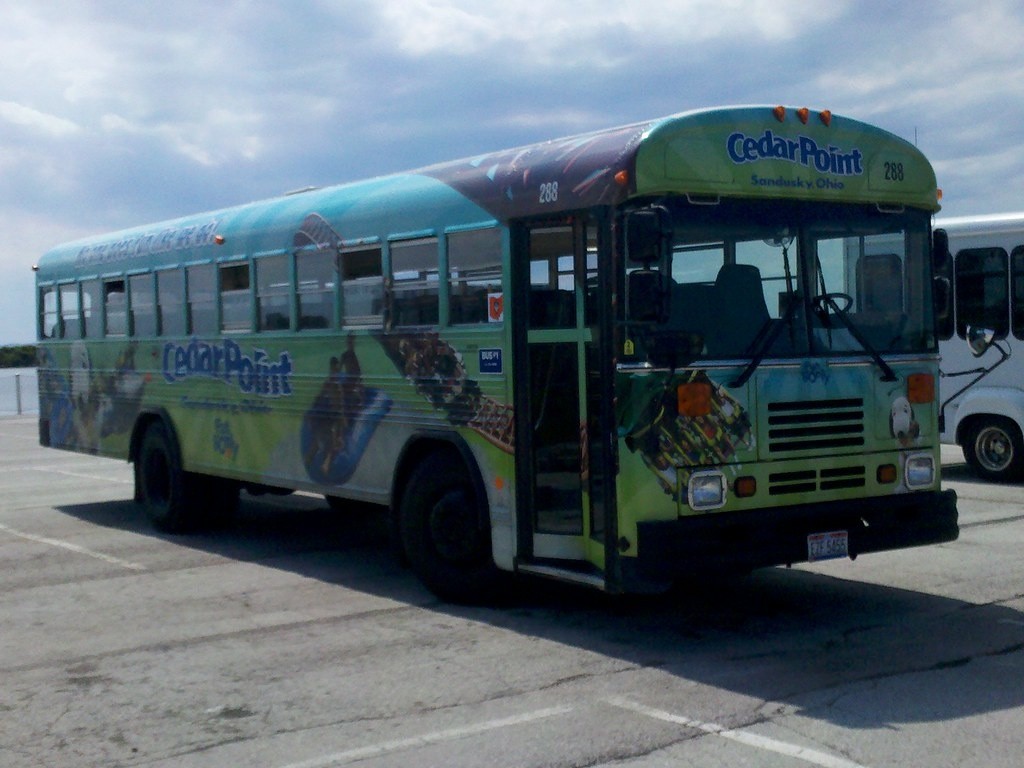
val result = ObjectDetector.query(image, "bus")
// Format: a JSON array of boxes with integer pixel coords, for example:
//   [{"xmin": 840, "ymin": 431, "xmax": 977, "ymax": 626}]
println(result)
[
  {"xmin": 844, "ymin": 211, "xmax": 1024, "ymax": 483},
  {"xmin": 34, "ymin": 104, "xmax": 1013, "ymax": 600}
]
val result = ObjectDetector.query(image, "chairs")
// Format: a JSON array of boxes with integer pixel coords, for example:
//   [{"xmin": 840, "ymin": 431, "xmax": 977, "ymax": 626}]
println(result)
[
  {"xmin": 630, "ymin": 266, "xmax": 666, "ymax": 323},
  {"xmin": 711, "ymin": 263, "xmax": 772, "ymax": 358}
]
